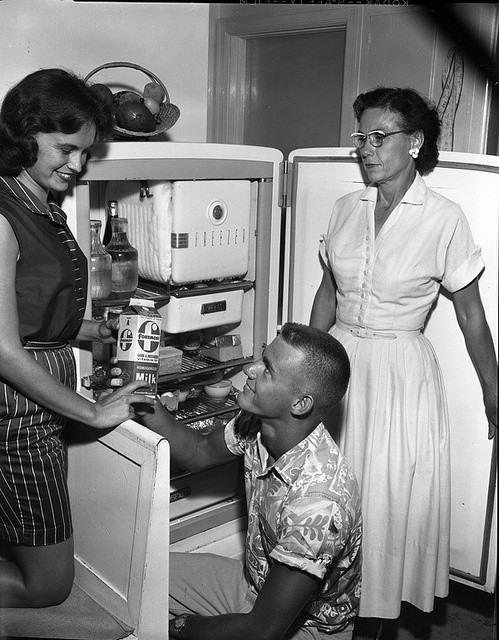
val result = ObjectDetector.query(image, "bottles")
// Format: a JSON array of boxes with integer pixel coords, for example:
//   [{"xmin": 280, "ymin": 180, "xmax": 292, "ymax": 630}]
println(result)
[
  {"xmin": 102, "ymin": 200, "xmax": 119, "ymax": 247},
  {"xmin": 104, "ymin": 218, "xmax": 138, "ymax": 300},
  {"xmin": 90, "ymin": 220, "xmax": 112, "ymax": 301}
]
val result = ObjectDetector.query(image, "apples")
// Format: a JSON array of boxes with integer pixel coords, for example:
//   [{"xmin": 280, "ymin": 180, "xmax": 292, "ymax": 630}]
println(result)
[{"xmin": 145, "ymin": 82, "xmax": 165, "ymax": 103}]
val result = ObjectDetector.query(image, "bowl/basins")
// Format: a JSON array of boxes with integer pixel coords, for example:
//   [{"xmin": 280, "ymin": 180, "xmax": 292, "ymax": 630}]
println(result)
[{"xmin": 204, "ymin": 379, "xmax": 232, "ymax": 403}]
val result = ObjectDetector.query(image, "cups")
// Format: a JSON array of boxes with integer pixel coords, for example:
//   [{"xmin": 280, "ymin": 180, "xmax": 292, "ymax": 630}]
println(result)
[
  {"xmin": 157, "ymin": 389, "xmax": 180, "ymax": 411},
  {"xmin": 169, "ymin": 385, "xmax": 195, "ymax": 402}
]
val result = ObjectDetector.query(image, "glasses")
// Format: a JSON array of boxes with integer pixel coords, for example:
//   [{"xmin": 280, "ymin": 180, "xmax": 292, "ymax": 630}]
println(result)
[{"xmin": 350, "ymin": 130, "xmax": 405, "ymax": 150}]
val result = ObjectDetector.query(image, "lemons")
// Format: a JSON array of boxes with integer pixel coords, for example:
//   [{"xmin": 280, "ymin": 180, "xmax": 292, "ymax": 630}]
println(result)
[{"xmin": 143, "ymin": 97, "xmax": 160, "ymax": 114}]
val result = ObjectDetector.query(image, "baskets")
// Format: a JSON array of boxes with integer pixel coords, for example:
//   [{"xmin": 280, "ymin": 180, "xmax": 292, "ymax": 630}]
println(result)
[{"xmin": 82, "ymin": 62, "xmax": 180, "ymax": 143}]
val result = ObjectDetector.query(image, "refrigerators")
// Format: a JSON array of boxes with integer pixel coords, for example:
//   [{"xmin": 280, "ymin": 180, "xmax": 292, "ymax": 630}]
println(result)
[{"xmin": 61, "ymin": 142, "xmax": 499, "ymax": 620}]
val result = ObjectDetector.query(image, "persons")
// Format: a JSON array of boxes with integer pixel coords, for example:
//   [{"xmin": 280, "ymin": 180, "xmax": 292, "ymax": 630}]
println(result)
[
  {"xmin": 106, "ymin": 322, "xmax": 364, "ymax": 640},
  {"xmin": 0, "ymin": 67, "xmax": 157, "ymax": 609},
  {"xmin": 309, "ymin": 87, "xmax": 499, "ymax": 638}
]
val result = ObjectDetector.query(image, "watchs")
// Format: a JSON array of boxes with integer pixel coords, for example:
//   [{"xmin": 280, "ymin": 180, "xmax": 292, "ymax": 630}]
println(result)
[{"xmin": 173, "ymin": 613, "xmax": 189, "ymax": 640}]
